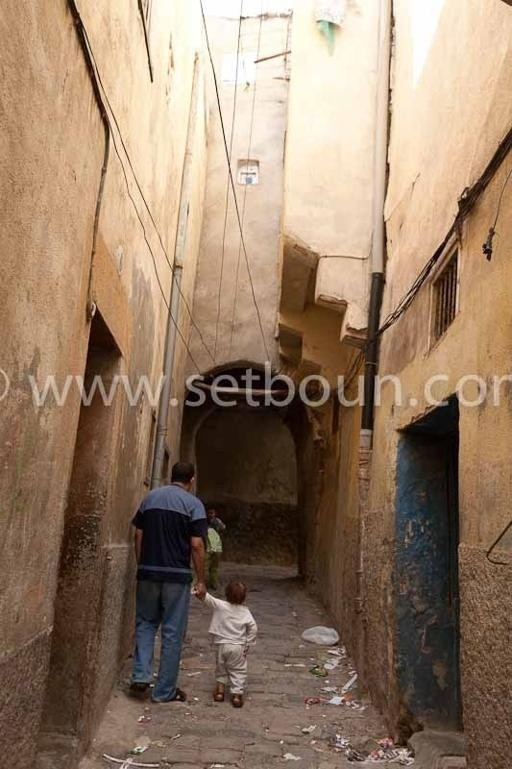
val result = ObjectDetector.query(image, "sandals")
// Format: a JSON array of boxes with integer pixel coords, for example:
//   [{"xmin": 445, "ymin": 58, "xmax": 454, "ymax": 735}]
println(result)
[
  {"xmin": 232, "ymin": 693, "xmax": 243, "ymax": 707},
  {"xmin": 212, "ymin": 688, "xmax": 225, "ymax": 701}
]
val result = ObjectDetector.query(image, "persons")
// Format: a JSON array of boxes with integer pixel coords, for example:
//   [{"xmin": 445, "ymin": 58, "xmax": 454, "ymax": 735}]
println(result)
[
  {"xmin": 190, "ymin": 527, "xmax": 224, "ymax": 591},
  {"xmin": 128, "ymin": 460, "xmax": 209, "ymax": 705},
  {"xmin": 206, "ymin": 506, "xmax": 226, "ymax": 530},
  {"xmin": 193, "ymin": 582, "xmax": 261, "ymax": 707}
]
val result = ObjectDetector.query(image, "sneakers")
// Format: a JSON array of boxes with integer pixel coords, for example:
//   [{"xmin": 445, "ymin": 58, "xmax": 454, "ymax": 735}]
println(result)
[
  {"xmin": 205, "ymin": 583, "xmax": 220, "ymax": 589},
  {"xmin": 129, "ymin": 681, "xmax": 147, "ymax": 691},
  {"xmin": 153, "ymin": 686, "xmax": 187, "ymax": 703}
]
